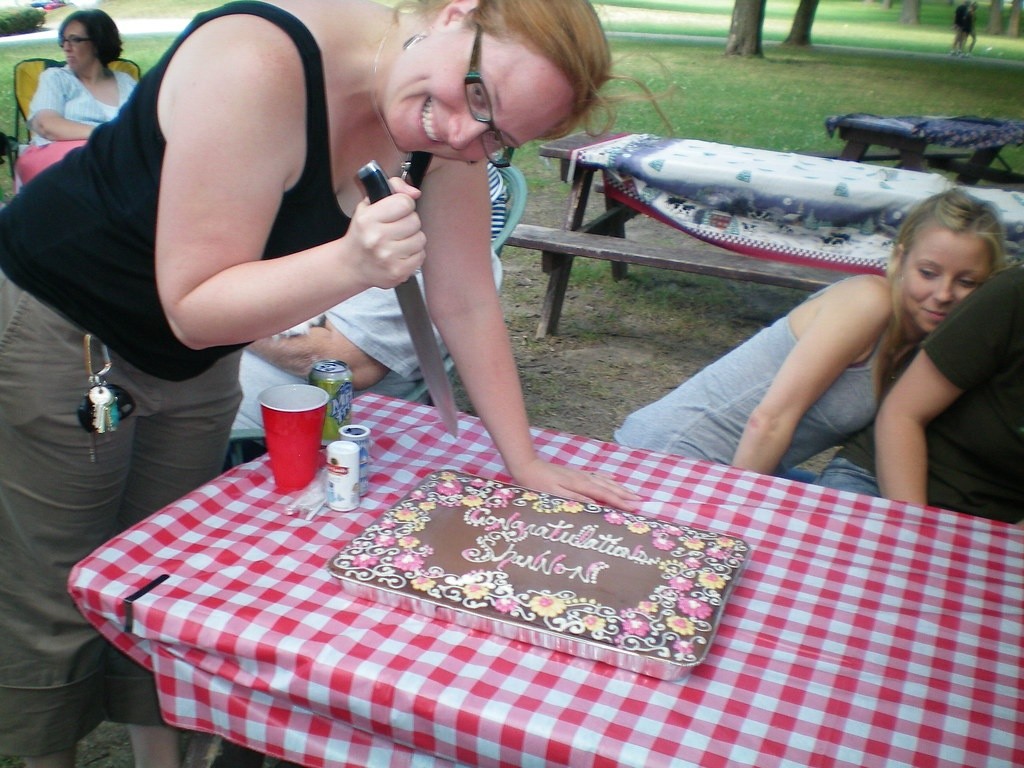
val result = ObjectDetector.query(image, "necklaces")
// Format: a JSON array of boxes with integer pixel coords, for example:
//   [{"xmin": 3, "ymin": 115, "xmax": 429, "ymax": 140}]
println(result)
[
  {"xmin": 890, "ymin": 372, "xmax": 897, "ymax": 382},
  {"xmin": 369, "ymin": 32, "xmax": 412, "ymax": 185}
]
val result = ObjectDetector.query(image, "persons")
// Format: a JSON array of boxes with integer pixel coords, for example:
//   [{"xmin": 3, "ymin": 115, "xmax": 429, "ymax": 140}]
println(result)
[
  {"xmin": 231, "ymin": 157, "xmax": 511, "ymax": 434},
  {"xmin": 13, "ymin": 8, "xmax": 138, "ymax": 194},
  {"xmin": 950, "ymin": 0, "xmax": 979, "ymax": 58},
  {"xmin": 611, "ymin": 184, "xmax": 1005, "ymax": 486},
  {"xmin": 809, "ymin": 249, "xmax": 1023, "ymax": 531},
  {"xmin": 0, "ymin": 0, "xmax": 608, "ymax": 768}
]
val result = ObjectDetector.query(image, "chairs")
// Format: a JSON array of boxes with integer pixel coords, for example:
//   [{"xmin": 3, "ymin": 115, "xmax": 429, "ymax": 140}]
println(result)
[
  {"xmin": 0, "ymin": 58, "xmax": 141, "ymax": 181},
  {"xmin": 229, "ymin": 151, "xmax": 527, "ymax": 466}
]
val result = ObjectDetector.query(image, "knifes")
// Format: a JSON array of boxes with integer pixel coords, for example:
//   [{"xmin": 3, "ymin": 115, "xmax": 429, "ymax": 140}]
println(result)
[{"xmin": 356, "ymin": 159, "xmax": 458, "ymax": 443}]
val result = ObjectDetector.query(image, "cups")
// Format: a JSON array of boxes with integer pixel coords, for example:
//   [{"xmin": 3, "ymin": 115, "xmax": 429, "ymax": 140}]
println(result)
[{"xmin": 257, "ymin": 384, "xmax": 329, "ymax": 493}]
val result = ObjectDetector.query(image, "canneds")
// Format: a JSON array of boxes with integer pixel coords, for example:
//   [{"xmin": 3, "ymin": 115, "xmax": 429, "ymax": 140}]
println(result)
[{"xmin": 307, "ymin": 359, "xmax": 353, "ymax": 446}]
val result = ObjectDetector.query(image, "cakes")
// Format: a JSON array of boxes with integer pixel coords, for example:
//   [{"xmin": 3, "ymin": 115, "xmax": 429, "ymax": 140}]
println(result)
[{"xmin": 329, "ymin": 469, "xmax": 747, "ymax": 664}]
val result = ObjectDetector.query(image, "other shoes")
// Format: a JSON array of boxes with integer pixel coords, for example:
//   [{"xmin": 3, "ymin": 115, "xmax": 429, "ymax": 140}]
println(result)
[{"xmin": 951, "ymin": 50, "xmax": 973, "ymax": 57}]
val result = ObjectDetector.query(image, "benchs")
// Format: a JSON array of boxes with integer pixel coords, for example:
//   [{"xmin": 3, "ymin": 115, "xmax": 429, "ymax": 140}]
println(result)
[
  {"xmin": 800, "ymin": 145, "xmax": 973, "ymax": 174},
  {"xmin": 505, "ymin": 223, "xmax": 855, "ymax": 292}
]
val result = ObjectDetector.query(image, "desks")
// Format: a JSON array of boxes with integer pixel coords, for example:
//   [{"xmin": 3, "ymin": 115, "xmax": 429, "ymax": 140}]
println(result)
[
  {"xmin": 535, "ymin": 129, "xmax": 1024, "ymax": 343},
  {"xmin": 824, "ymin": 113, "xmax": 1024, "ymax": 188},
  {"xmin": 68, "ymin": 391, "xmax": 1024, "ymax": 768}
]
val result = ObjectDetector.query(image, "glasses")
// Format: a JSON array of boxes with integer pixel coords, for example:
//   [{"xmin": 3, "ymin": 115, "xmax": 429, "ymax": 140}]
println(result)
[
  {"xmin": 463, "ymin": 23, "xmax": 515, "ymax": 169},
  {"xmin": 57, "ymin": 35, "xmax": 91, "ymax": 47}
]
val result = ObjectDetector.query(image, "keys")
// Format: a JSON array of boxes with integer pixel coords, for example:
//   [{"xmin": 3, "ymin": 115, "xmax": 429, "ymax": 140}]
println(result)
[{"xmin": 77, "ymin": 384, "xmax": 135, "ymax": 462}]
